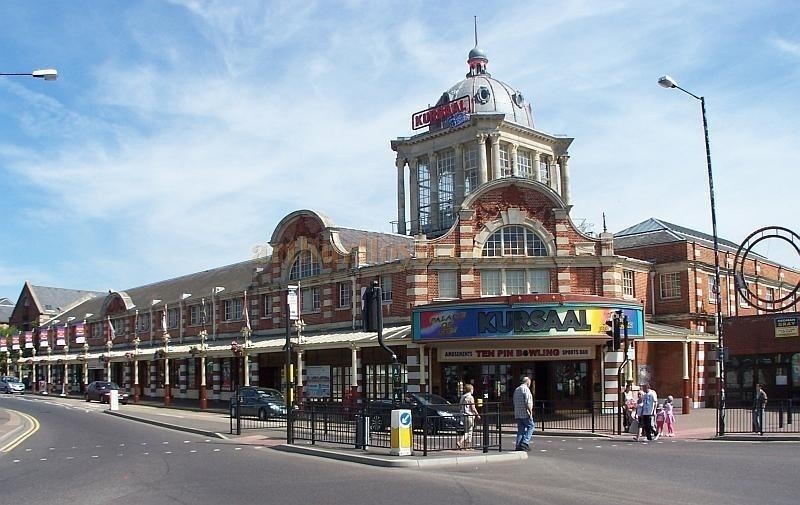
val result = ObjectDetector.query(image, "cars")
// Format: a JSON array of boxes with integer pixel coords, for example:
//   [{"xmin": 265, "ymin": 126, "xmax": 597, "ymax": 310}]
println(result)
[
  {"xmin": 367, "ymin": 393, "xmax": 464, "ymax": 436},
  {"xmin": 0, "ymin": 376, "xmax": 26, "ymax": 395},
  {"xmin": 83, "ymin": 381, "xmax": 128, "ymax": 405},
  {"xmin": 229, "ymin": 386, "xmax": 299, "ymax": 422}
]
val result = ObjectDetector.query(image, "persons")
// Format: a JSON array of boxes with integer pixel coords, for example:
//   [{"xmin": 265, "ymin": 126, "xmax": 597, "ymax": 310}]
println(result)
[
  {"xmin": 512, "ymin": 377, "xmax": 535, "ymax": 450},
  {"xmin": 455, "ymin": 384, "xmax": 481, "ymax": 451},
  {"xmin": 622, "ymin": 381, "xmax": 675, "ymax": 442},
  {"xmin": 752, "ymin": 383, "xmax": 767, "ymax": 432}
]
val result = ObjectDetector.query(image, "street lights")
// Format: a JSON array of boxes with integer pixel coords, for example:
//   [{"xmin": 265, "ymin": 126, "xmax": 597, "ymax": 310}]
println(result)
[
  {"xmin": 0, "ymin": 69, "xmax": 58, "ymax": 81},
  {"xmin": 656, "ymin": 74, "xmax": 729, "ymax": 436}
]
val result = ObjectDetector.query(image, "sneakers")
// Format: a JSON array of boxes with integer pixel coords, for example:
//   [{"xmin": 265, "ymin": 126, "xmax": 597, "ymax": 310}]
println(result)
[
  {"xmin": 633, "ymin": 433, "xmax": 674, "ymax": 441},
  {"xmin": 456, "ymin": 441, "xmax": 474, "ymax": 450},
  {"xmin": 516, "ymin": 445, "xmax": 530, "ymax": 451}
]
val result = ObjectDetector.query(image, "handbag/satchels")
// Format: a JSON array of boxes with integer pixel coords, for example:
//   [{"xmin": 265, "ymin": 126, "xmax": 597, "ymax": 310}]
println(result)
[{"xmin": 628, "ymin": 419, "xmax": 638, "ymax": 433}]
